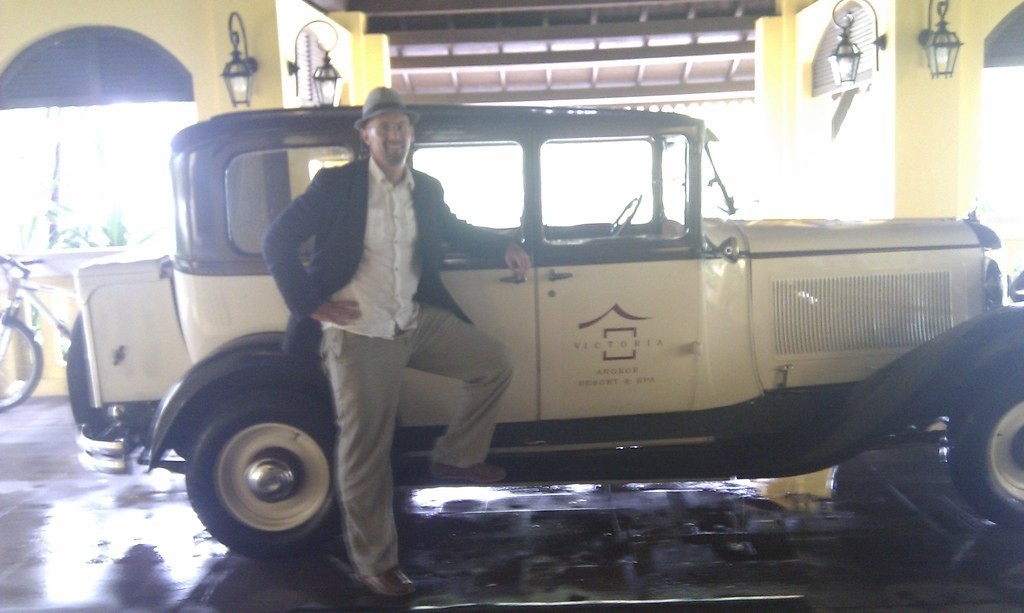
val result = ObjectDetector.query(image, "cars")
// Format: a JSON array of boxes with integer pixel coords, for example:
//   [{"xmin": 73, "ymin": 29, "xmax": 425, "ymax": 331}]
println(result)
[{"xmin": 64, "ymin": 102, "xmax": 1023, "ymax": 559}]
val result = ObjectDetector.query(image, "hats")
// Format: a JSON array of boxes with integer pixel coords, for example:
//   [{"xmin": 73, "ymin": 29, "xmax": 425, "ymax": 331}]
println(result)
[{"xmin": 354, "ymin": 88, "xmax": 421, "ymax": 130}]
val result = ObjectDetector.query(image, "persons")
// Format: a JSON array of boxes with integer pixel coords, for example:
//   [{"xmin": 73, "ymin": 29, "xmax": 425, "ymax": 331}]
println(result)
[{"xmin": 261, "ymin": 85, "xmax": 532, "ymax": 598}]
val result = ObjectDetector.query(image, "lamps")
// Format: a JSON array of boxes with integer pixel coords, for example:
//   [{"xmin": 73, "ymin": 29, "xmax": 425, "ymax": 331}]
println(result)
[
  {"xmin": 287, "ymin": 20, "xmax": 344, "ymax": 107},
  {"xmin": 219, "ymin": 11, "xmax": 257, "ymax": 108},
  {"xmin": 827, "ymin": 0, "xmax": 887, "ymax": 87},
  {"xmin": 918, "ymin": 0, "xmax": 964, "ymax": 79}
]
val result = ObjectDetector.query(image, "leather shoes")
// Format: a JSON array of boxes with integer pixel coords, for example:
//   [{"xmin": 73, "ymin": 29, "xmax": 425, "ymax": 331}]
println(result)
[
  {"xmin": 349, "ymin": 568, "xmax": 417, "ymax": 600},
  {"xmin": 428, "ymin": 459, "xmax": 507, "ymax": 483}
]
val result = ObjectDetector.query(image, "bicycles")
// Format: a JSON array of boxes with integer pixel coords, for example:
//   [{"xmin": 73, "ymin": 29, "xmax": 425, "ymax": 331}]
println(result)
[{"xmin": 0, "ymin": 253, "xmax": 72, "ymax": 416}]
